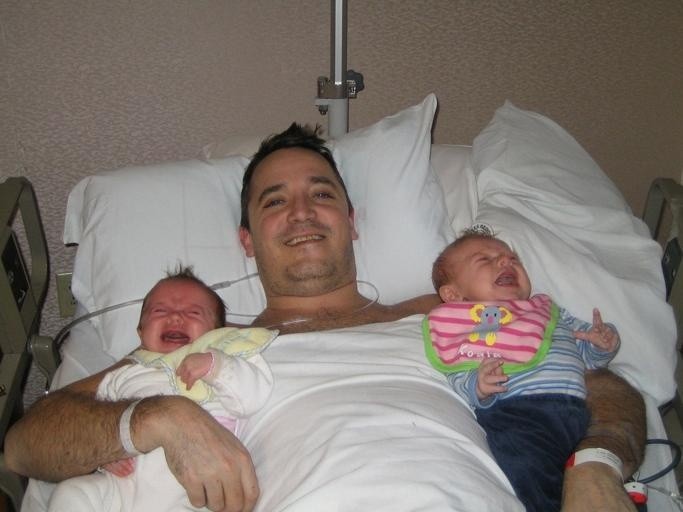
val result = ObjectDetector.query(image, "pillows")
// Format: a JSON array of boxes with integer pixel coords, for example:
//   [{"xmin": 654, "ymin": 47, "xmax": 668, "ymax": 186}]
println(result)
[
  {"xmin": 60, "ymin": 94, "xmax": 457, "ymax": 364},
  {"xmin": 430, "ymin": 144, "xmax": 475, "ymax": 239}
]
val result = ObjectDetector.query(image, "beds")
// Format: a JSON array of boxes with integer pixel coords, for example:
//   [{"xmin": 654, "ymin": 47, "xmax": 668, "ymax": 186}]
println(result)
[{"xmin": 0, "ymin": 174, "xmax": 683, "ymax": 509}]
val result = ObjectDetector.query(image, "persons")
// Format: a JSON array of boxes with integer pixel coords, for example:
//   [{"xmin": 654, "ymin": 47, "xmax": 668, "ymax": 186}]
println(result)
[
  {"xmin": 48, "ymin": 273, "xmax": 274, "ymax": 512},
  {"xmin": 4, "ymin": 123, "xmax": 647, "ymax": 512},
  {"xmin": 423, "ymin": 233, "xmax": 648, "ymax": 512}
]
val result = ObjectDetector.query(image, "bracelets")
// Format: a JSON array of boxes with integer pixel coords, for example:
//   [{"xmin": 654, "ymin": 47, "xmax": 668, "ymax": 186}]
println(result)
[
  {"xmin": 562, "ymin": 448, "xmax": 624, "ymax": 480},
  {"xmin": 118, "ymin": 399, "xmax": 142, "ymax": 458}
]
